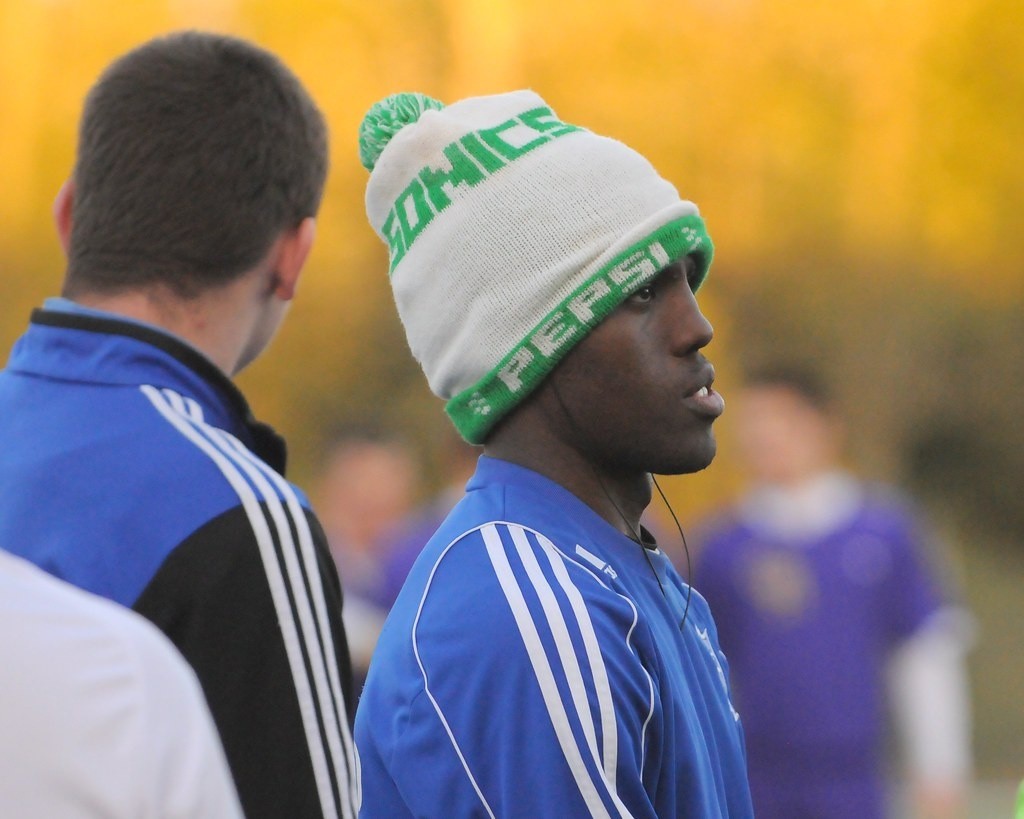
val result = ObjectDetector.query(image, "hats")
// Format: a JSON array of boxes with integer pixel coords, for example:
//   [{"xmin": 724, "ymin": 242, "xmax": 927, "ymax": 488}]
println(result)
[{"xmin": 358, "ymin": 87, "xmax": 717, "ymax": 445}]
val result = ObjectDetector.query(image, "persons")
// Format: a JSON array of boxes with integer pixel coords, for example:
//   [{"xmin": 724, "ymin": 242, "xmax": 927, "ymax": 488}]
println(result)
[
  {"xmin": 0, "ymin": 546, "xmax": 243, "ymax": 819},
  {"xmin": 0, "ymin": 32, "xmax": 362, "ymax": 819},
  {"xmin": 300, "ymin": 417, "xmax": 502, "ymax": 673},
  {"xmin": 354, "ymin": 88, "xmax": 755, "ymax": 819},
  {"xmin": 672, "ymin": 360, "xmax": 977, "ymax": 819}
]
